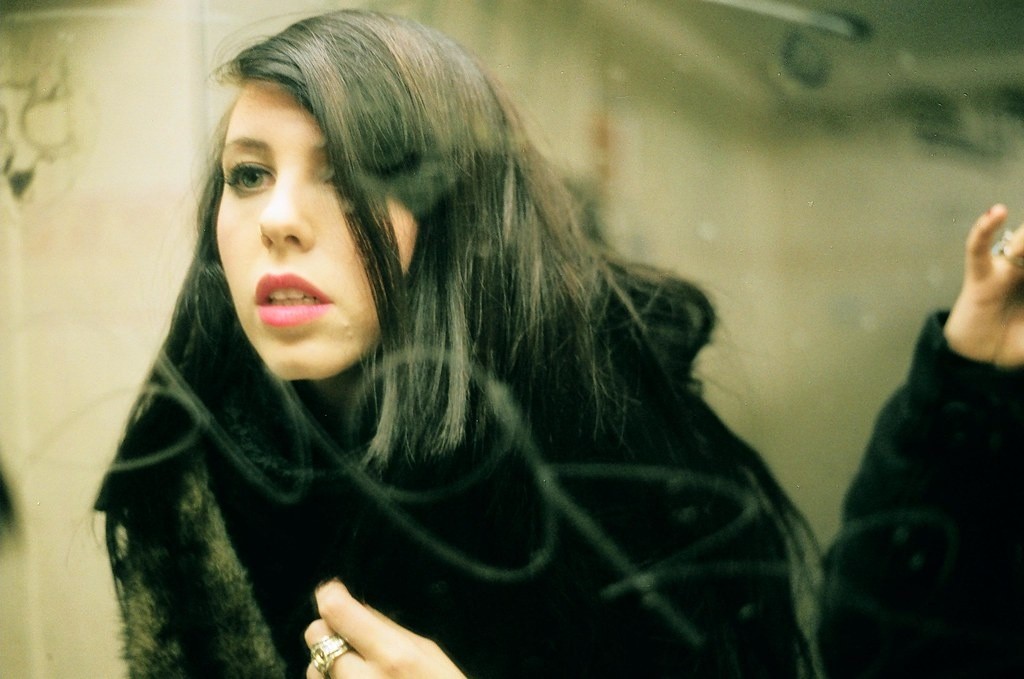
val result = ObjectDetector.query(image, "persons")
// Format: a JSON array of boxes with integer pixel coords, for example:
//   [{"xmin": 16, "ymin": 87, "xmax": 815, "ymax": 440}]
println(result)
[{"xmin": 88, "ymin": 0, "xmax": 1024, "ymax": 679}]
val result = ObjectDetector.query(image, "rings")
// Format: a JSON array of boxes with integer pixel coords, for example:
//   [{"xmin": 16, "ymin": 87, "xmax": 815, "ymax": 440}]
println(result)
[{"xmin": 311, "ymin": 633, "xmax": 354, "ymax": 679}]
